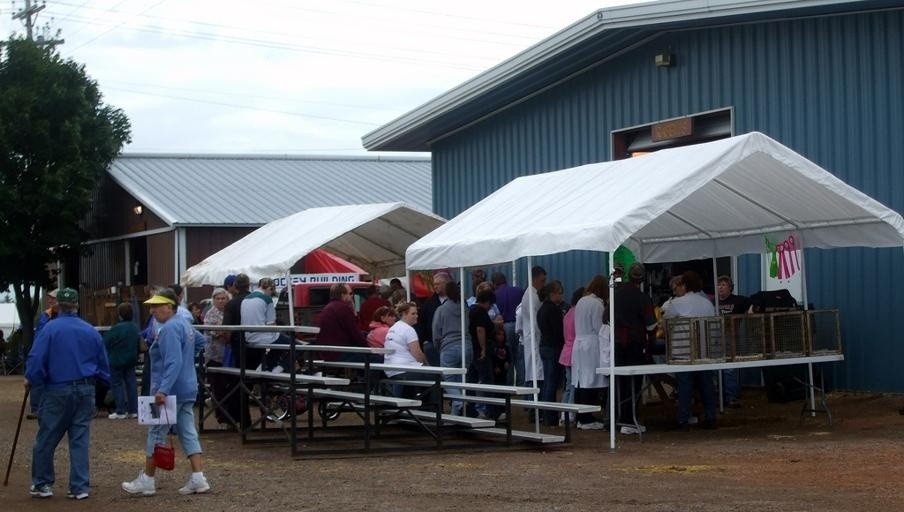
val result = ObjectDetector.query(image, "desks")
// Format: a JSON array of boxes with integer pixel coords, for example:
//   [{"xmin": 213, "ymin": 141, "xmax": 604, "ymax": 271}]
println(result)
[{"xmin": 593, "ymin": 352, "xmax": 847, "ymax": 443}]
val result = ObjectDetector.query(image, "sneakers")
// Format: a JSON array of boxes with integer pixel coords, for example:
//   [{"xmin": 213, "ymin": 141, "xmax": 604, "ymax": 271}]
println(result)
[
  {"xmin": 67, "ymin": 489, "xmax": 89, "ymax": 499},
  {"xmin": 108, "ymin": 412, "xmax": 128, "ymax": 419},
  {"xmin": 122, "ymin": 472, "xmax": 156, "ymax": 496},
  {"xmin": 577, "ymin": 421, "xmax": 604, "ymax": 430},
  {"xmin": 179, "ymin": 477, "xmax": 210, "ymax": 494},
  {"xmin": 620, "ymin": 424, "xmax": 647, "ymax": 434},
  {"xmin": 559, "ymin": 418, "xmax": 576, "ymax": 428},
  {"xmin": 30, "ymin": 484, "xmax": 53, "ymax": 497},
  {"xmin": 129, "ymin": 413, "xmax": 138, "ymax": 420}
]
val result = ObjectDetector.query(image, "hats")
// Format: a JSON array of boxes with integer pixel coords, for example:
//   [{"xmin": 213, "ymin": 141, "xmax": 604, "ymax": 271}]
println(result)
[
  {"xmin": 259, "ymin": 278, "xmax": 278, "ymax": 297},
  {"xmin": 235, "ymin": 273, "xmax": 250, "ymax": 286},
  {"xmin": 628, "ymin": 262, "xmax": 645, "ymax": 279},
  {"xmin": 225, "ymin": 275, "xmax": 237, "ymax": 284},
  {"xmin": 56, "ymin": 287, "xmax": 79, "ymax": 302},
  {"xmin": 143, "ymin": 294, "xmax": 177, "ymax": 307},
  {"xmin": 47, "ymin": 289, "xmax": 60, "ymax": 298}
]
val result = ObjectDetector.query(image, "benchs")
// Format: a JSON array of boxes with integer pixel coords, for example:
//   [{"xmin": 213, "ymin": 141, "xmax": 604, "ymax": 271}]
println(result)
[{"xmin": 87, "ymin": 320, "xmax": 603, "ymax": 450}]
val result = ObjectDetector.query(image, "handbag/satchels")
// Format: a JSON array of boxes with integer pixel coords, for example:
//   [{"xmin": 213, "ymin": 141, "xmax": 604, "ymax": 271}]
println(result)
[{"xmin": 152, "ymin": 442, "xmax": 175, "ymax": 471}]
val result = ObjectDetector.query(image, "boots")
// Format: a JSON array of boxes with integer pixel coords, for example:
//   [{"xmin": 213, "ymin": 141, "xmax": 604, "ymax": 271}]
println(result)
[{"xmin": 26, "ymin": 405, "xmax": 37, "ymax": 419}]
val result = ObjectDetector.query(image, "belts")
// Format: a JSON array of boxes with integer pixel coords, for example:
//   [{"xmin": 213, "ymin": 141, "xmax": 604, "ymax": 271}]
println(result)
[{"xmin": 69, "ymin": 379, "xmax": 95, "ymax": 386}]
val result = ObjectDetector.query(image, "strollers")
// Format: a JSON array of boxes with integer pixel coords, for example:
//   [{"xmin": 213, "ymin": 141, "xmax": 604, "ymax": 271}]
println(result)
[{"xmin": 259, "ymin": 336, "xmax": 342, "ymax": 423}]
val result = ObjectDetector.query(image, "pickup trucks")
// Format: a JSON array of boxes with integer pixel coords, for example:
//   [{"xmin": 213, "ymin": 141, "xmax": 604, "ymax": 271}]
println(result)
[{"xmin": 276, "ymin": 281, "xmax": 390, "ymax": 340}]
